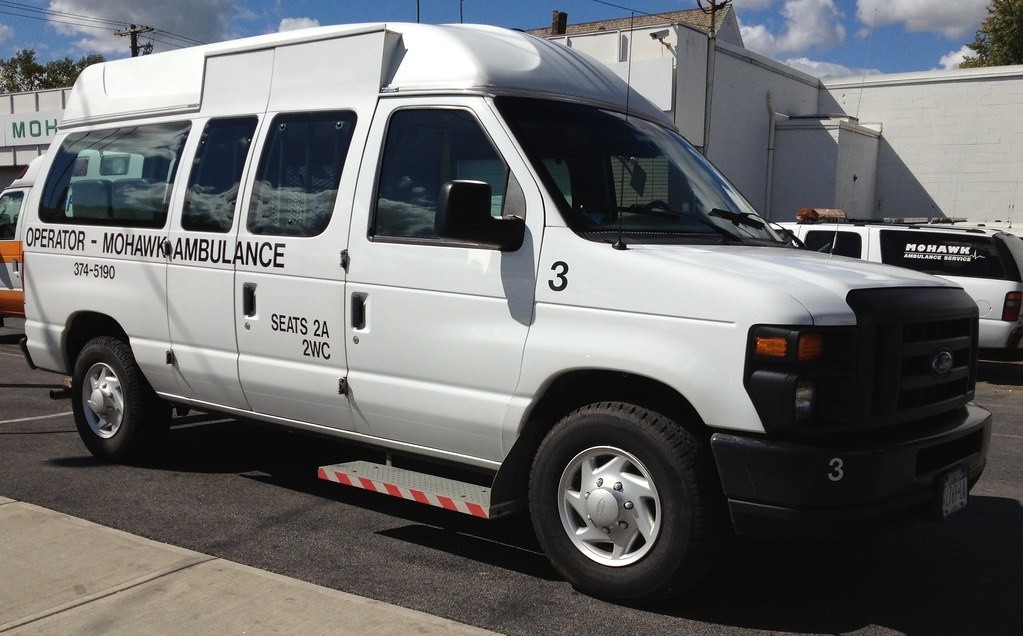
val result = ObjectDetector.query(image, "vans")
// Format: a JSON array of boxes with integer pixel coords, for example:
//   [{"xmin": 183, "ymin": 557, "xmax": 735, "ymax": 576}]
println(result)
[
  {"xmin": 0, "ymin": 152, "xmax": 51, "ymax": 318},
  {"xmin": 17, "ymin": 17, "xmax": 994, "ymax": 601},
  {"xmin": 768, "ymin": 207, "xmax": 1022, "ymax": 354}
]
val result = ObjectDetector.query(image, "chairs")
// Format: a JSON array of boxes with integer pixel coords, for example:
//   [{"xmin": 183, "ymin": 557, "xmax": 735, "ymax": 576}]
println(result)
[
  {"xmin": 72, "ymin": 179, "xmax": 112, "ymax": 219},
  {"xmin": 113, "ymin": 178, "xmax": 156, "ymax": 220}
]
